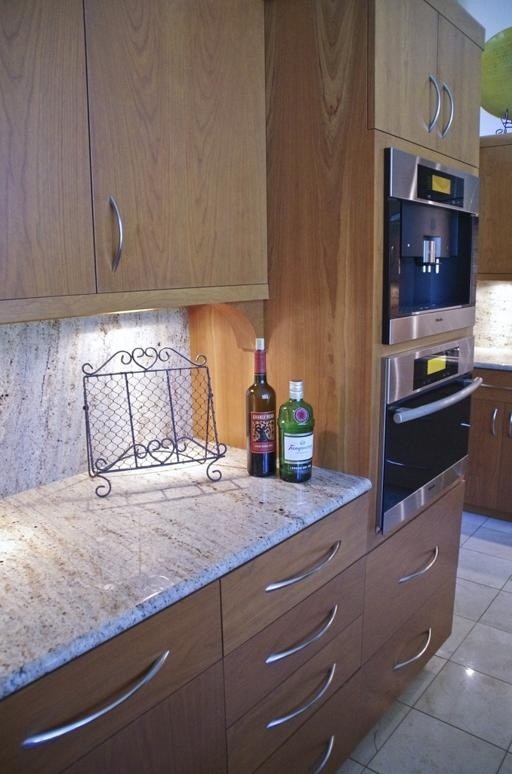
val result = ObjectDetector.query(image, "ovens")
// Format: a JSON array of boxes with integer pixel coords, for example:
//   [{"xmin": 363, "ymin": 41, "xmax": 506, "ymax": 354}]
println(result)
[{"xmin": 375, "ymin": 145, "xmax": 484, "ymax": 539}]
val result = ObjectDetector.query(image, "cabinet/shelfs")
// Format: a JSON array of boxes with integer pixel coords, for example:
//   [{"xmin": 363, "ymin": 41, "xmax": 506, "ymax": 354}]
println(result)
[
  {"xmin": 266, "ymin": 1, "xmax": 483, "ymax": 168},
  {"xmin": 1, "ymin": 0, "xmax": 272, "ymax": 325},
  {"xmin": 368, "ymin": 476, "xmax": 468, "ymax": 722},
  {"xmin": 221, "ymin": 453, "xmax": 370, "ymax": 771},
  {"xmin": 0, "ymin": 578, "xmax": 218, "ymax": 773},
  {"xmin": 463, "ymin": 376, "xmax": 512, "ymax": 517},
  {"xmin": 474, "ymin": 131, "xmax": 511, "ymax": 279}
]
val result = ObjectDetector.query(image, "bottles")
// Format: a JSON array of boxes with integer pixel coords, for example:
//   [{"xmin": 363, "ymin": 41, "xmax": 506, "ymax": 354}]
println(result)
[
  {"xmin": 277, "ymin": 379, "xmax": 315, "ymax": 485},
  {"xmin": 244, "ymin": 338, "xmax": 278, "ymax": 477}
]
